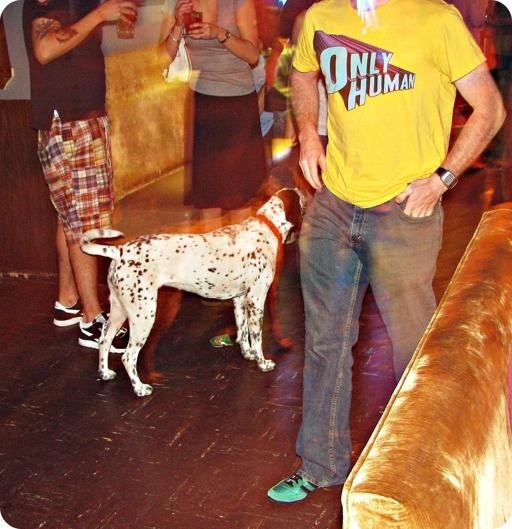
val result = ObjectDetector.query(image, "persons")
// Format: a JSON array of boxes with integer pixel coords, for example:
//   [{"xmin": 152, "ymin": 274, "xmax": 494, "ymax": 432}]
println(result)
[
  {"xmin": 275, "ymin": 0, "xmax": 328, "ymax": 152},
  {"xmin": 265, "ymin": 0, "xmax": 506, "ymax": 505},
  {"xmin": 446, "ymin": 0, "xmax": 503, "ymax": 174},
  {"xmin": 18, "ymin": 0, "xmax": 138, "ymax": 354},
  {"xmin": 158, "ymin": 0, "xmax": 269, "ymax": 308}
]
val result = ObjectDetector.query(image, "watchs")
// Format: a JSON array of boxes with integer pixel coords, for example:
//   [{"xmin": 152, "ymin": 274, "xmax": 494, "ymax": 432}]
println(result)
[
  {"xmin": 436, "ymin": 165, "xmax": 458, "ymax": 189},
  {"xmin": 218, "ymin": 27, "xmax": 231, "ymax": 43}
]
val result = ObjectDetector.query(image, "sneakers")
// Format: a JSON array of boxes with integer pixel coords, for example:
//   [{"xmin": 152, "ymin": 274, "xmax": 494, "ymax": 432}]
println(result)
[
  {"xmin": 267, "ymin": 472, "xmax": 318, "ymax": 504},
  {"xmin": 79, "ymin": 312, "xmax": 129, "ymax": 353},
  {"xmin": 52, "ymin": 300, "xmax": 84, "ymax": 327}
]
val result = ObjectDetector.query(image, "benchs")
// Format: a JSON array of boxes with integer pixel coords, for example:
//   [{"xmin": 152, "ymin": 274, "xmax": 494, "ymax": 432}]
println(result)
[
  {"xmin": 339, "ymin": 207, "xmax": 512, "ymax": 529},
  {"xmin": 104, "ymin": 44, "xmax": 293, "ymax": 243}
]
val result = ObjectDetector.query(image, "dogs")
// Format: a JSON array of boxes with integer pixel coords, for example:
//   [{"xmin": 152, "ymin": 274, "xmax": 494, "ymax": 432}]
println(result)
[{"xmin": 78, "ymin": 186, "xmax": 308, "ymax": 399}]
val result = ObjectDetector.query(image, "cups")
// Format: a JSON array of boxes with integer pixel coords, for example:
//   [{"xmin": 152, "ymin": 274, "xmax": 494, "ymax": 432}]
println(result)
[
  {"xmin": 182, "ymin": 12, "xmax": 204, "ymax": 34},
  {"xmin": 115, "ymin": 6, "xmax": 137, "ymax": 40}
]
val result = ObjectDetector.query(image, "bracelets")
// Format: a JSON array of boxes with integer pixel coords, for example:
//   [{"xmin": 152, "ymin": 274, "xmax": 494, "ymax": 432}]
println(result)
[{"xmin": 167, "ymin": 30, "xmax": 177, "ymax": 42}]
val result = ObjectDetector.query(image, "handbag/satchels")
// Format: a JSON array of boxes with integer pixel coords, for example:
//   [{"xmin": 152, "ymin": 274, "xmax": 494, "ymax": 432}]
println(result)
[{"xmin": 162, "ymin": 36, "xmax": 191, "ymax": 83}]
